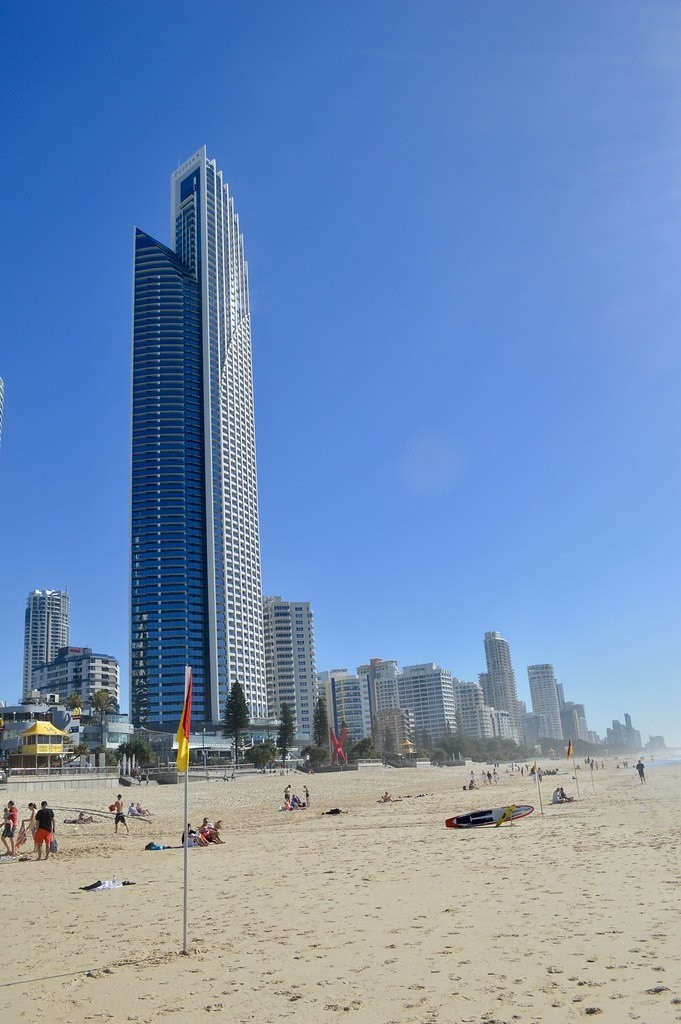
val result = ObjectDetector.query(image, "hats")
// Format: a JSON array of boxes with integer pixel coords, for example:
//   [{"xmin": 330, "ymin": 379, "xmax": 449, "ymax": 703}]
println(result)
[
  {"xmin": 203, "ymin": 829, "xmax": 209, "ymax": 835},
  {"xmin": 117, "ymin": 795, "xmax": 121, "ymax": 798}
]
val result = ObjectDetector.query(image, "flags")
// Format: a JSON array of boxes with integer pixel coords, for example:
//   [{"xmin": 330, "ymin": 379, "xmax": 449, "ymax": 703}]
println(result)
[
  {"xmin": 175, "ymin": 669, "xmax": 192, "ymax": 773},
  {"xmin": 565, "ymin": 741, "xmax": 574, "ymax": 763}
]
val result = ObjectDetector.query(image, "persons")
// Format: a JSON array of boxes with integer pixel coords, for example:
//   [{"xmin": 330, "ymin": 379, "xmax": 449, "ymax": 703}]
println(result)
[
  {"xmin": 379, "ymin": 791, "xmax": 393, "ymax": 803},
  {"xmin": 231, "ymin": 774, "xmax": 236, "ymax": 781},
  {"xmin": 282, "ymin": 784, "xmax": 311, "ymax": 812},
  {"xmin": 181, "ymin": 817, "xmax": 226, "ymax": 848},
  {"xmin": 63, "ymin": 812, "xmax": 103, "ymax": 826},
  {"xmin": 206, "ymin": 770, "xmax": 210, "ymax": 783},
  {"xmin": 112, "ymin": 794, "xmax": 130, "ymax": 834},
  {"xmin": 127, "ymin": 802, "xmax": 152, "ymax": 816},
  {"xmin": 223, "ymin": 775, "xmax": 229, "ymax": 781},
  {"xmin": 468, "ymin": 754, "xmax": 654, "ymax": 804},
  {"xmin": 0, "ymin": 801, "xmax": 56, "ymax": 861}
]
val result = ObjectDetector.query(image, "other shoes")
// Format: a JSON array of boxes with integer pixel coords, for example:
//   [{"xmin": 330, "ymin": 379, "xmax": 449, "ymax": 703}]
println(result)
[{"xmin": 122, "ymin": 881, "xmax": 136, "ymax": 885}]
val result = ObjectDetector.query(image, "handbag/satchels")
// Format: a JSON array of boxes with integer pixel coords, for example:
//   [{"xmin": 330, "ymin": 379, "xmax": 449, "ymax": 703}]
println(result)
[
  {"xmin": 463, "ymin": 786, "xmax": 466, "ymax": 790},
  {"xmin": 50, "ymin": 840, "xmax": 58, "ymax": 853}
]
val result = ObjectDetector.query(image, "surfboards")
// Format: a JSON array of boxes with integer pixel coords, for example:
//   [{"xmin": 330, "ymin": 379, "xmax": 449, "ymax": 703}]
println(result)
[{"xmin": 445, "ymin": 805, "xmax": 535, "ymax": 828}]
